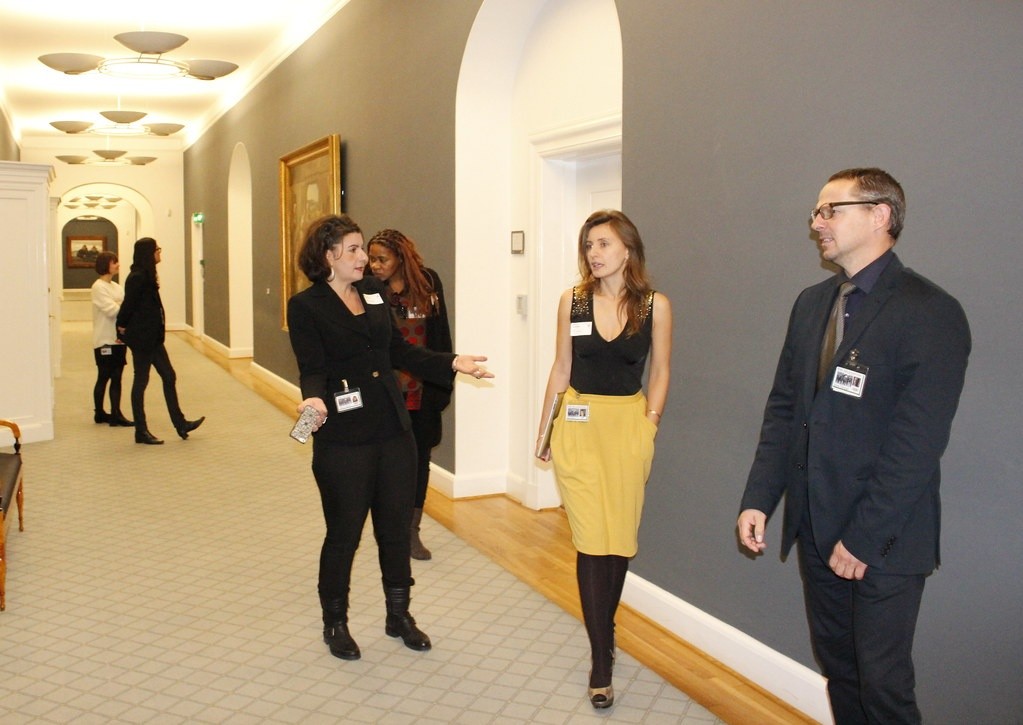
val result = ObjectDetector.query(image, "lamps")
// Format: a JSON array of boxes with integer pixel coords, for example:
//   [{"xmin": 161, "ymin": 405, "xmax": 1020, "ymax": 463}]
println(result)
[
  {"xmin": 50, "ymin": 110, "xmax": 184, "ymax": 138},
  {"xmin": 65, "ymin": 194, "xmax": 123, "ymax": 209},
  {"xmin": 57, "ymin": 149, "xmax": 158, "ymax": 166},
  {"xmin": 38, "ymin": 30, "xmax": 240, "ymax": 80}
]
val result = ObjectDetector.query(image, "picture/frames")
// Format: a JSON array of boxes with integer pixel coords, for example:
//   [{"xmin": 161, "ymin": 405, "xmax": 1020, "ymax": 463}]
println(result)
[
  {"xmin": 281, "ymin": 132, "xmax": 342, "ymax": 330},
  {"xmin": 66, "ymin": 234, "xmax": 108, "ymax": 267}
]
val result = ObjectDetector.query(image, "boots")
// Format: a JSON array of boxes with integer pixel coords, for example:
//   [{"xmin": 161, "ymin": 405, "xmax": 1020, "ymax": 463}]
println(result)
[
  {"xmin": 318, "ymin": 587, "xmax": 361, "ymax": 660},
  {"xmin": 410, "ymin": 508, "xmax": 432, "ymax": 560},
  {"xmin": 384, "ymin": 587, "xmax": 432, "ymax": 651}
]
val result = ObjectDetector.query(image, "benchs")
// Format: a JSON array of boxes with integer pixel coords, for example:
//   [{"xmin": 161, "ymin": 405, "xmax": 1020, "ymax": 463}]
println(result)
[{"xmin": 0, "ymin": 419, "xmax": 24, "ymax": 610}]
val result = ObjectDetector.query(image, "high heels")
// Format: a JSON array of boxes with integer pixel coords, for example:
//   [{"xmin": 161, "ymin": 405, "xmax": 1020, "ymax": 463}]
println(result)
[
  {"xmin": 588, "ymin": 647, "xmax": 614, "ymax": 708},
  {"xmin": 135, "ymin": 429, "xmax": 165, "ymax": 444},
  {"xmin": 176, "ymin": 416, "xmax": 205, "ymax": 440}
]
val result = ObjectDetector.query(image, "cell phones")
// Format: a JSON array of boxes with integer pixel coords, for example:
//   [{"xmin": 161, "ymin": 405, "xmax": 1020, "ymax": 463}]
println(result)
[{"xmin": 290, "ymin": 405, "xmax": 320, "ymax": 444}]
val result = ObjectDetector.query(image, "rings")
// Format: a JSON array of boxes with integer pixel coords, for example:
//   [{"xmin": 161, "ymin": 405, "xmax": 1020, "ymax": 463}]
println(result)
[{"xmin": 477, "ymin": 377, "xmax": 481, "ymax": 379}]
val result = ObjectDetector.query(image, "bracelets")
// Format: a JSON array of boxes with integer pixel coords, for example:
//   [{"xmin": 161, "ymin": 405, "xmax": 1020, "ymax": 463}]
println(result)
[
  {"xmin": 536, "ymin": 434, "xmax": 544, "ymax": 441},
  {"xmin": 452, "ymin": 356, "xmax": 458, "ymax": 372},
  {"xmin": 648, "ymin": 409, "xmax": 661, "ymax": 418}
]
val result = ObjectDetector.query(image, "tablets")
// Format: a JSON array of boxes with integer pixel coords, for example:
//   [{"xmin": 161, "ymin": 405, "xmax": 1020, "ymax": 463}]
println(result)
[{"xmin": 537, "ymin": 391, "xmax": 566, "ymax": 457}]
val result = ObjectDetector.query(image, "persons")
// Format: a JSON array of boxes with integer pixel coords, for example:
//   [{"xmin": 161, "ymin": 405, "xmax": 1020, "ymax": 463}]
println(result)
[
  {"xmin": 536, "ymin": 211, "xmax": 672, "ymax": 708},
  {"xmin": 580, "ymin": 410, "xmax": 585, "ymax": 416},
  {"xmin": 287, "ymin": 213, "xmax": 494, "ymax": 660},
  {"xmin": 363, "ymin": 229, "xmax": 453, "ymax": 560},
  {"xmin": 116, "ymin": 237, "xmax": 205, "ymax": 445},
  {"xmin": 91, "ymin": 252, "xmax": 135, "ymax": 427},
  {"xmin": 737, "ymin": 168, "xmax": 972, "ymax": 725}
]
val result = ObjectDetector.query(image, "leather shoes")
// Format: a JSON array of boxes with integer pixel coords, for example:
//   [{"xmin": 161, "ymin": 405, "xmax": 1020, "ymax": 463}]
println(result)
[
  {"xmin": 94, "ymin": 409, "xmax": 111, "ymax": 423},
  {"xmin": 110, "ymin": 409, "xmax": 135, "ymax": 426}
]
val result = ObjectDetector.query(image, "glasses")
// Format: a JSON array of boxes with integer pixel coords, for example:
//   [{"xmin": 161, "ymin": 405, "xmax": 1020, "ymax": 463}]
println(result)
[
  {"xmin": 155, "ymin": 246, "xmax": 162, "ymax": 252},
  {"xmin": 811, "ymin": 201, "xmax": 880, "ymax": 222}
]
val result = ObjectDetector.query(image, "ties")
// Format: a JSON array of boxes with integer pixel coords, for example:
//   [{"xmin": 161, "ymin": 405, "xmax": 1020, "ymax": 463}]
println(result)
[{"xmin": 816, "ymin": 282, "xmax": 856, "ymax": 391}]
